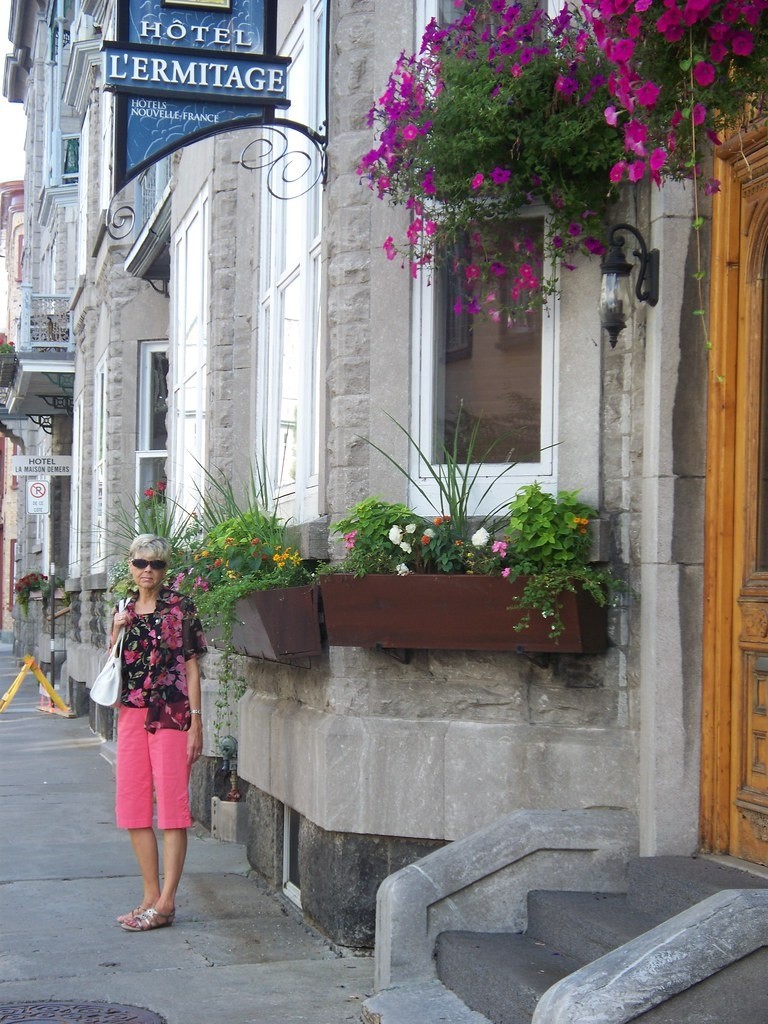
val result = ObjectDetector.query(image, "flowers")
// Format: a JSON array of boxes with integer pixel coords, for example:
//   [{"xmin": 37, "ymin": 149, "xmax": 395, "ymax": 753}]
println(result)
[
  {"xmin": 143, "ymin": 422, "xmax": 317, "ymax": 771},
  {"xmin": 14, "ymin": 571, "xmax": 49, "ymax": 622},
  {"xmin": 313, "ymin": 399, "xmax": 640, "ymax": 646},
  {"xmin": 0, "ymin": 339, "xmax": 16, "ymax": 355}
]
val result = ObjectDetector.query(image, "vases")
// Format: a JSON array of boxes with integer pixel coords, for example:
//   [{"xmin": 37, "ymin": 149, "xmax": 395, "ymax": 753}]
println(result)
[
  {"xmin": 0, "ymin": 353, "xmax": 18, "ymax": 387},
  {"xmin": 29, "ymin": 590, "xmax": 43, "ymax": 601},
  {"xmin": 319, "ymin": 570, "xmax": 608, "ymax": 654},
  {"xmin": 198, "ymin": 583, "xmax": 323, "ymax": 662}
]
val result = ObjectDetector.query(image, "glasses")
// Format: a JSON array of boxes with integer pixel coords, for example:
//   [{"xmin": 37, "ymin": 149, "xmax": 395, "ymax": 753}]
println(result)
[{"xmin": 129, "ymin": 558, "xmax": 167, "ymax": 570}]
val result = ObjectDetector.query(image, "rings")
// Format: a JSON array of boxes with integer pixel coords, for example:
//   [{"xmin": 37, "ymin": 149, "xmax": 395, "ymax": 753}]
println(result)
[
  {"xmin": 198, "ymin": 753, "xmax": 201, "ymax": 755},
  {"xmin": 119, "ymin": 616, "xmax": 122, "ymax": 620}
]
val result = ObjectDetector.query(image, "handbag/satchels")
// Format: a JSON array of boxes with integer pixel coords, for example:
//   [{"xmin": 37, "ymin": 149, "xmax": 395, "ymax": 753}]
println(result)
[{"xmin": 90, "ymin": 596, "xmax": 134, "ymax": 707}]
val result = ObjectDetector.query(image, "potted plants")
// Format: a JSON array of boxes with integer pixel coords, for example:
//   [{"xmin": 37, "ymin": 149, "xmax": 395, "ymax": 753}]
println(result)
[{"xmin": 53, "ymin": 574, "xmax": 71, "ymax": 607}]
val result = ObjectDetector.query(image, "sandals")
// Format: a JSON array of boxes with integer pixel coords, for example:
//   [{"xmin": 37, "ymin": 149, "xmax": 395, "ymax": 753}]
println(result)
[
  {"xmin": 116, "ymin": 906, "xmax": 149, "ymax": 925},
  {"xmin": 121, "ymin": 907, "xmax": 176, "ymax": 932}
]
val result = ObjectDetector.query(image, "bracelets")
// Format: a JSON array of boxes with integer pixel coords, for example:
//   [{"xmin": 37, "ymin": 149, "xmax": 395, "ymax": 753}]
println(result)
[{"xmin": 190, "ymin": 709, "xmax": 201, "ymax": 714}]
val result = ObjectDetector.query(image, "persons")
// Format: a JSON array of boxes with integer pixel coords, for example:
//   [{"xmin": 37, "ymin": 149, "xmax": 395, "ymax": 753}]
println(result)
[{"xmin": 108, "ymin": 534, "xmax": 208, "ymax": 933}]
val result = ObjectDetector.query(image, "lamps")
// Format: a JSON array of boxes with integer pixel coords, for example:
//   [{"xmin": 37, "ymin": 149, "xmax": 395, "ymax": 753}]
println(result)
[{"xmin": 599, "ymin": 222, "xmax": 661, "ymax": 350}]
